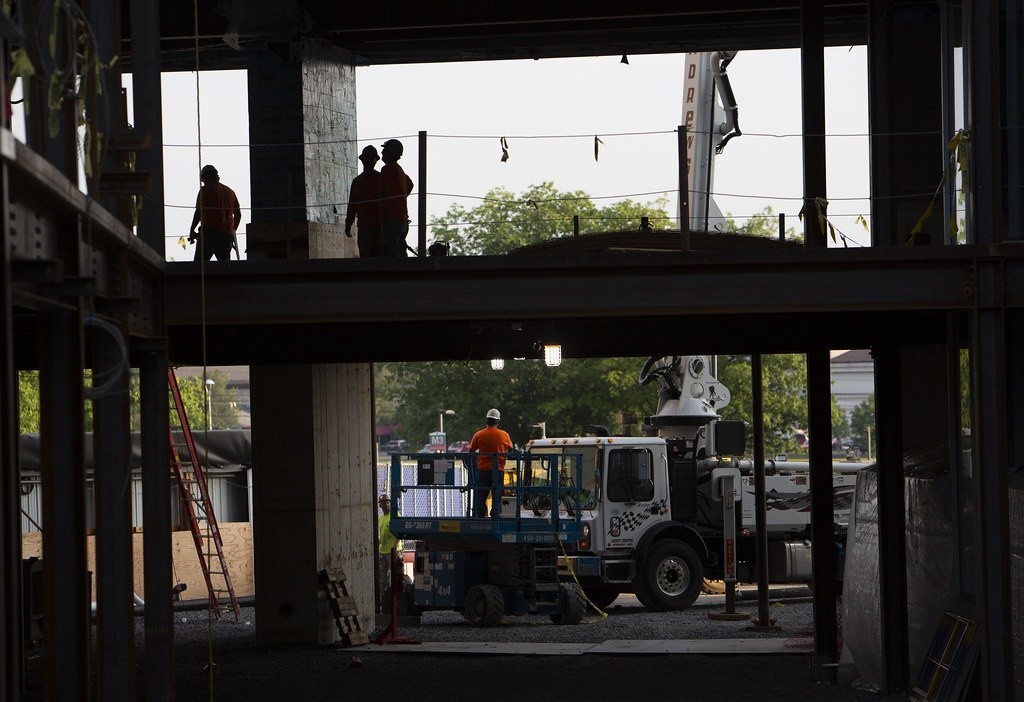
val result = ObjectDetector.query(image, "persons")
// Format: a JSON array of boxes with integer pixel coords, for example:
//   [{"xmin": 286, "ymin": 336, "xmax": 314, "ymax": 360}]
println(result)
[
  {"xmin": 378, "ymin": 494, "xmax": 404, "ymax": 600},
  {"xmin": 189, "ymin": 165, "xmax": 241, "ymax": 262},
  {"xmin": 469, "ymin": 409, "xmax": 513, "ymax": 518},
  {"xmin": 345, "ymin": 145, "xmax": 381, "ymax": 256},
  {"xmin": 375, "ymin": 139, "xmax": 413, "ymax": 257}
]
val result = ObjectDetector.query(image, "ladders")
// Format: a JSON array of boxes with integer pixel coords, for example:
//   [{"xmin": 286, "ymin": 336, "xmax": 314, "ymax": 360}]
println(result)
[{"xmin": 168, "ymin": 367, "xmax": 242, "ymax": 621}]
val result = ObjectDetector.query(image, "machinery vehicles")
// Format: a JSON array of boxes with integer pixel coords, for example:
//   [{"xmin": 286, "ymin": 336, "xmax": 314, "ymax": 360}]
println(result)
[
  {"xmin": 527, "ymin": 51, "xmax": 878, "ymax": 613},
  {"xmin": 382, "ymin": 451, "xmax": 604, "ymax": 621}
]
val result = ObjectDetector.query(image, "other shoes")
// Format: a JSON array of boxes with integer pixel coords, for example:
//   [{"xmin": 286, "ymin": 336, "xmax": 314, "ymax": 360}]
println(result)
[
  {"xmin": 478, "ymin": 513, "xmax": 485, "ymax": 518},
  {"xmin": 491, "ymin": 513, "xmax": 501, "ymax": 518}
]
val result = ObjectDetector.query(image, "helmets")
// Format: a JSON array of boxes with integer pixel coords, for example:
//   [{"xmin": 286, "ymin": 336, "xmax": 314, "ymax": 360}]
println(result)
[
  {"xmin": 359, "ymin": 145, "xmax": 380, "ymax": 161},
  {"xmin": 378, "ymin": 494, "xmax": 390, "ymax": 502},
  {"xmin": 381, "ymin": 139, "xmax": 403, "ymax": 156},
  {"xmin": 485, "ymin": 408, "xmax": 501, "ymax": 420},
  {"xmin": 201, "ymin": 165, "xmax": 217, "ymax": 178}
]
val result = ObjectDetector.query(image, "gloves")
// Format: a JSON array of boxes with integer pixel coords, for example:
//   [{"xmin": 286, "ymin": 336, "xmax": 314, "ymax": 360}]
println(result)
[{"xmin": 345, "ymin": 223, "xmax": 352, "ymax": 237}]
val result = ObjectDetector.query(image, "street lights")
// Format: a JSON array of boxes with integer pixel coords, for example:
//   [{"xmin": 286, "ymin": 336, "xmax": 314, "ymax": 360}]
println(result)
[
  {"xmin": 202, "ymin": 378, "xmax": 216, "ymax": 430},
  {"xmin": 438, "ymin": 408, "xmax": 456, "ymax": 433}
]
